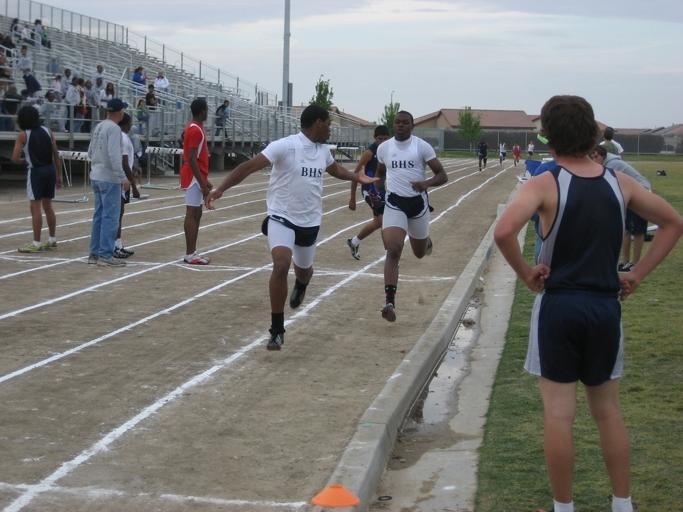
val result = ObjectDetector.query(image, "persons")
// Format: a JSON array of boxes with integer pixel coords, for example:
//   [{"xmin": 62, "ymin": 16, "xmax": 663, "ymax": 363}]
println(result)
[
  {"xmin": 0, "ymin": 18, "xmax": 52, "ymax": 128},
  {"xmin": 179, "ymin": 99, "xmax": 213, "ymax": 264},
  {"xmin": 589, "ymin": 145, "xmax": 653, "ymax": 272},
  {"xmin": 493, "ymin": 94, "xmax": 683, "ymax": 512},
  {"xmin": 346, "ymin": 125, "xmax": 435, "ymax": 260},
  {"xmin": 131, "ymin": 65, "xmax": 229, "ymax": 144},
  {"xmin": 601, "ymin": 125, "xmax": 623, "ymax": 158},
  {"xmin": 203, "ymin": 104, "xmax": 382, "ymax": 351},
  {"xmin": 52, "ymin": 69, "xmax": 133, "ymax": 133},
  {"xmin": 87, "ymin": 98, "xmax": 130, "ymax": 266},
  {"xmin": 114, "ymin": 111, "xmax": 140, "ymax": 258},
  {"xmin": 12, "ymin": 105, "xmax": 62, "ymax": 254},
  {"xmin": 473, "ymin": 136, "xmax": 534, "ymax": 172},
  {"xmin": 374, "ymin": 110, "xmax": 448, "ymax": 322}
]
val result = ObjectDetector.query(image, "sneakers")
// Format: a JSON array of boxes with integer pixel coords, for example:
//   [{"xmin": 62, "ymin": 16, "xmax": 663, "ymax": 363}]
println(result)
[
  {"xmin": 18, "ymin": 239, "xmax": 210, "ymax": 268},
  {"xmin": 619, "ymin": 262, "xmax": 634, "ymax": 271},
  {"xmin": 266, "ymin": 238, "xmax": 432, "ymax": 351}
]
